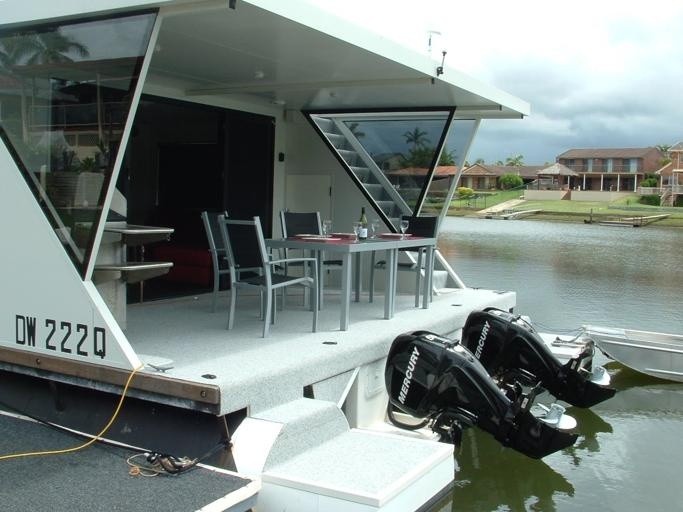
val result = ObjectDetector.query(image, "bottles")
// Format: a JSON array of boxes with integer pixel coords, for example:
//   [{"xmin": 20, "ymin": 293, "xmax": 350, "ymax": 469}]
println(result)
[{"xmin": 358, "ymin": 207, "xmax": 368, "ymax": 240}]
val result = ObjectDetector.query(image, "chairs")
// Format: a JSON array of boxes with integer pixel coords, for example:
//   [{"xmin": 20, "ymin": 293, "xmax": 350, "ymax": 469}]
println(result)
[{"xmin": 202, "ymin": 209, "xmax": 440, "ymax": 339}]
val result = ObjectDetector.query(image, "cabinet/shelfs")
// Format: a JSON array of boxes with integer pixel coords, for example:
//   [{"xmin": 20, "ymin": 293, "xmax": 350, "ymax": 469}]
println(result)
[{"xmin": 72, "ymin": 221, "xmax": 175, "ymax": 284}]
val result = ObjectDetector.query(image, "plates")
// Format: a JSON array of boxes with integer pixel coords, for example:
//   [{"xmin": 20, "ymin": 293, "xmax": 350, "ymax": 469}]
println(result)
[
  {"xmin": 295, "ymin": 234, "xmax": 331, "ymax": 238},
  {"xmin": 301, "ymin": 237, "xmax": 341, "ymax": 241},
  {"xmin": 381, "ymin": 233, "xmax": 412, "ymax": 237},
  {"xmin": 332, "ymin": 232, "xmax": 356, "ymax": 237}
]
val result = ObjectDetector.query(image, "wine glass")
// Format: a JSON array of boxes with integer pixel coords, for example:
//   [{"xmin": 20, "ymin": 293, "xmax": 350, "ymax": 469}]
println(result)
[
  {"xmin": 370, "ymin": 219, "xmax": 380, "ymax": 240},
  {"xmin": 322, "ymin": 219, "xmax": 332, "ymax": 241},
  {"xmin": 350, "ymin": 221, "xmax": 363, "ymax": 243},
  {"xmin": 399, "ymin": 220, "xmax": 410, "ymax": 240}
]
